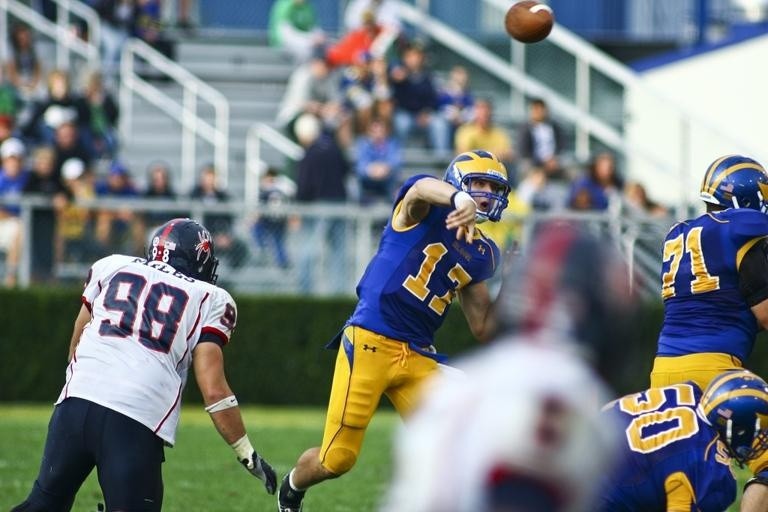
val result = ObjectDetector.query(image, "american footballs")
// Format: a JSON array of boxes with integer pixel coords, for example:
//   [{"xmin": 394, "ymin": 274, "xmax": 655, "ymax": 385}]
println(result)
[{"xmin": 506, "ymin": 1, "xmax": 556, "ymax": 43}]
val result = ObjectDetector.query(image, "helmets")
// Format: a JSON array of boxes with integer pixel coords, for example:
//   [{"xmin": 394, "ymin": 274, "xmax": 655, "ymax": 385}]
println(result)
[
  {"xmin": 698, "ymin": 367, "xmax": 767, "ymax": 466},
  {"xmin": 441, "ymin": 149, "xmax": 513, "ymax": 222},
  {"xmin": 699, "ymin": 152, "xmax": 767, "ymax": 212},
  {"xmin": 145, "ymin": 216, "xmax": 219, "ymax": 283}
]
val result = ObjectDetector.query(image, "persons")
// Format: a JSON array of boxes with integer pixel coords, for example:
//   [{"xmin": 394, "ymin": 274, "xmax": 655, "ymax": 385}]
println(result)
[
  {"xmin": 586, "ymin": 371, "xmax": 767, "ymax": 510},
  {"xmin": 278, "ymin": 152, "xmax": 511, "ymax": 510},
  {"xmin": 0, "ymin": 0, "xmax": 666, "ymax": 283},
  {"xmin": 376, "ymin": 218, "xmax": 635, "ymax": 511},
  {"xmin": 11, "ymin": 218, "xmax": 278, "ymax": 512},
  {"xmin": 646, "ymin": 153, "xmax": 767, "ymax": 510}
]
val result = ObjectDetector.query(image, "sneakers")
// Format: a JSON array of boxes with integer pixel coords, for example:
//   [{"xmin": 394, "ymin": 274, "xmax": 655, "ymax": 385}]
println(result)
[{"xmin": 275, "ymin": 470, "xmax": 307, "ymax": 511}]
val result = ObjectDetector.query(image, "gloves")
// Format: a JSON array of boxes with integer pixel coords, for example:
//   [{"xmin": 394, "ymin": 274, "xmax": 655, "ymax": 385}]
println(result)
[{"xmin": 231, "ymin": 434, "xmax": 277, "ymax": 494}]
